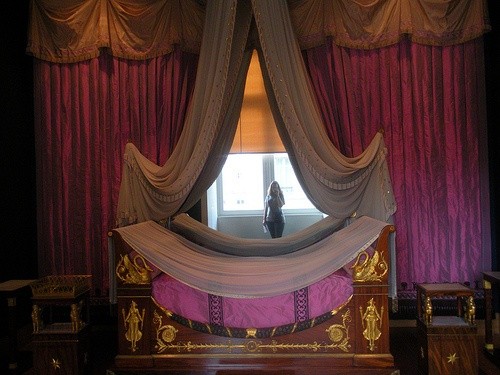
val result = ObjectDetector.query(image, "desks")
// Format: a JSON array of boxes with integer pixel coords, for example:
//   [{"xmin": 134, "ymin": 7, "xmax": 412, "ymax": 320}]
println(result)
[{"xmin": 482, "ymin": 271, "xmax": 500, "ymax": 357}]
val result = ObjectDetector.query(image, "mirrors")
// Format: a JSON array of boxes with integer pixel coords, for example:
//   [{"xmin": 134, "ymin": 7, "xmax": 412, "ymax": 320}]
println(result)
[{"xmin": 172, "ymin": 49, "xmax": 346, "ymax": 257}]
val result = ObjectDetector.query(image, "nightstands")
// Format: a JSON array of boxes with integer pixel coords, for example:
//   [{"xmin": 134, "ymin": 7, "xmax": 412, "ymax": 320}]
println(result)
[
  {"xmin": 417, "ymin": 283, "xmax": 479, "ymax": 375},
  {"xmin": 30, "ymin": 275, "xmax": 90, "ymax": 375}
]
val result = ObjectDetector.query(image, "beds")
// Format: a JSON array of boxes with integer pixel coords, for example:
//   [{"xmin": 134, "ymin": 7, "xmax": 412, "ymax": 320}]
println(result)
[{"xmin": 108, "ymin": 215, "xmax": 394, "ymax": 375}]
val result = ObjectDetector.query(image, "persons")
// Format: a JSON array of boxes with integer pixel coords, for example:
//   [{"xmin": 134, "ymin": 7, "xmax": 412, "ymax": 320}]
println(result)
[{"xmin": 262, "ymin": 182, "xmax": 286, "ymax": 238}]
